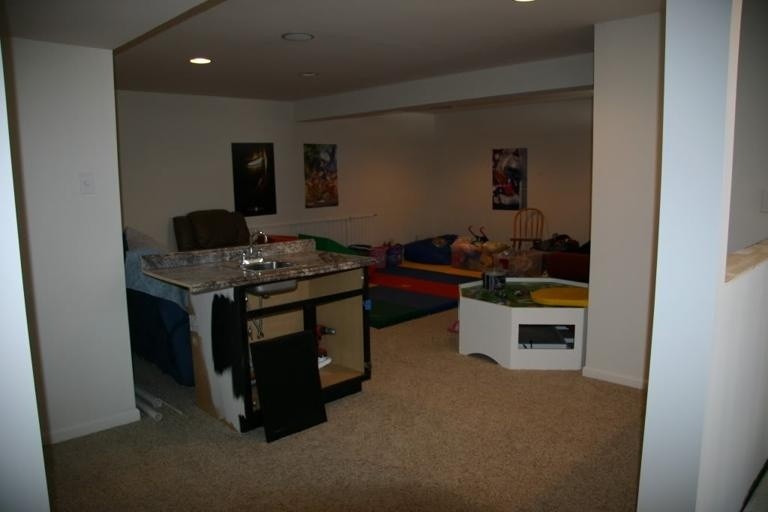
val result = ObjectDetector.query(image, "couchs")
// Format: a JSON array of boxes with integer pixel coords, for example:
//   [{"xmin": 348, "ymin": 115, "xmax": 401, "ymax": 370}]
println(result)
[
  {"xmin": 172, "ymin": 208, "xmax": 251, "ymax": 252},
  {"xmin": 402, "ymin": 234, "xmax": 510, "ymax": 273}
]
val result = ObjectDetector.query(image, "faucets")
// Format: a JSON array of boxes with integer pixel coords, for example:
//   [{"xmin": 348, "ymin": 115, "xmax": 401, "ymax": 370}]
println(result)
[{"xmin": 249, "ymin": 232, "xmax": 268, "ymax": 255}]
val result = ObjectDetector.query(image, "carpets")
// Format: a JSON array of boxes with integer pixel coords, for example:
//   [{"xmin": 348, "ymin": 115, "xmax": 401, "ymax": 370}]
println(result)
[{"xmin": 364, "ymin": 259, "xmax": 482, "ymax": 330}]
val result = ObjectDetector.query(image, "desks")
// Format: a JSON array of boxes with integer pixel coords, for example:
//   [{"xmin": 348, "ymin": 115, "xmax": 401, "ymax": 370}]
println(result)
[
  {"xmin": 457, "ymin": 276, "xmax": 588, "ymax": 371},
  {"xmin": 498, "ymin": 248, "xmax": 545, "ymax": 275}
]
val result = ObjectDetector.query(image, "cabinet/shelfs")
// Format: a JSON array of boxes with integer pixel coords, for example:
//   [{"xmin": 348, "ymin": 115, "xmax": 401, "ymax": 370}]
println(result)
[{"xmin": 186, "ymin": 266, "xmax": 370, "ymax": 433}]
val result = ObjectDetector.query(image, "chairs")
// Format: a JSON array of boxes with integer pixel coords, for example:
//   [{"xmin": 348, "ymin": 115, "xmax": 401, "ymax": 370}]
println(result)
[{"xmin": 510, "ymin": 207, "xmax": 545, "ymax": 252}]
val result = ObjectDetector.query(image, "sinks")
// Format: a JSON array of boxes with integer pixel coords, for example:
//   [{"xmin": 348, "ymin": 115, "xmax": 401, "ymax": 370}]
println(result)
[{"xmin": 242, "ymin": 261, "xmax": 294, "ymax": 271}]
